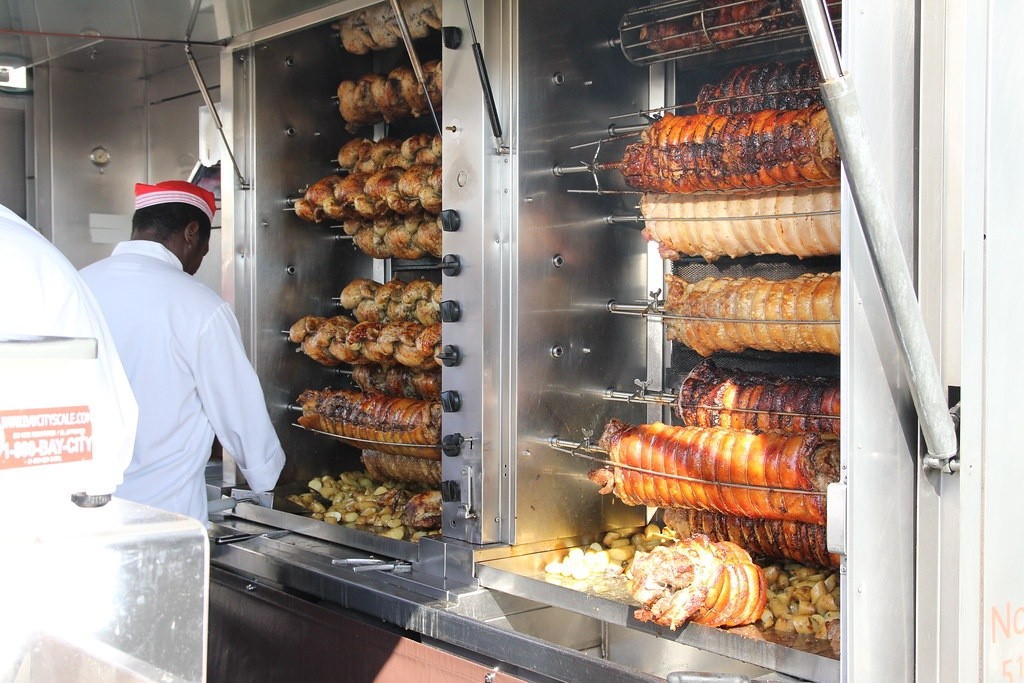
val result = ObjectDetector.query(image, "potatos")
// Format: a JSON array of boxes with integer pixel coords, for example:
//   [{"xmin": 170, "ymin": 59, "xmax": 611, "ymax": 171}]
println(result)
[
  {"xmin": 544, "ymin": 525, "xmax": 842, "ymax": 640},
  {"xmin": 287, "ymin": 469, "xmax": 444, "ymax": 540}
]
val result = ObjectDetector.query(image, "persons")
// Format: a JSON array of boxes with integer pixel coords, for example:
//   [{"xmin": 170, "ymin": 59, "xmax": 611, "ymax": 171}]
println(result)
[{"xmin": 79, "ymin": 180, "xmax": 286, "ymax": 530}]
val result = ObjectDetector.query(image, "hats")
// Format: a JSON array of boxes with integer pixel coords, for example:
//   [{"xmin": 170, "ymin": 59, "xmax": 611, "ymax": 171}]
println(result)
[{"xmin": 135, "ymin": 180, "xmax": 217, "ymax": 221}]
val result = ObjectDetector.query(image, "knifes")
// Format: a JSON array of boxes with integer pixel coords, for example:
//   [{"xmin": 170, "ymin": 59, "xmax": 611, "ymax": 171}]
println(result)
[{"xmin": 215, "ymin": 530, "xmax": 289, "ymax": 544}]
